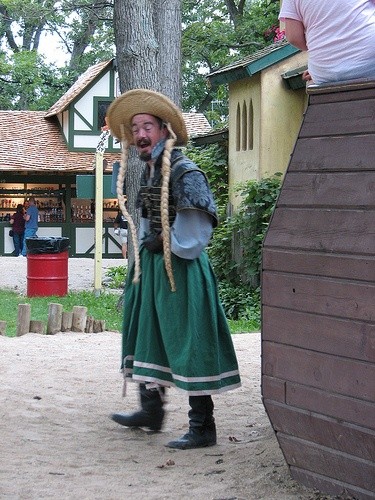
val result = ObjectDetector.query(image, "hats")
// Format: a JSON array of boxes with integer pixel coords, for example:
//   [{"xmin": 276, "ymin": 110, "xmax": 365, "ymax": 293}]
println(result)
[{"xmin": 107, "ymin": 89, "xmax": 188, "ymax": 146}]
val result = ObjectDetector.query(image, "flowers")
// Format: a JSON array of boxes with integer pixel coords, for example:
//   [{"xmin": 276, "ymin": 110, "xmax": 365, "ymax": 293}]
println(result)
[{"xmin": 273, "ymin": 28, "xmax": 285, "ymax": 44}]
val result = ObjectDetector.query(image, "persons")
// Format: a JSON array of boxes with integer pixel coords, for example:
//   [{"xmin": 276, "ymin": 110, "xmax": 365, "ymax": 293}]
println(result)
[
  {"xmin": 18, "ymin": 197, "xmax": 39, "ymax": 258},
  {"xmin": 277, "ymin": 0, "xmax": 375, "ymax": 85},
  {"xmin": 113, "ymin": 201, "xmax": 129, "ymax": 260},
  {"xmin": 107, "ymin": 89, "xmax": 242, "ymax": 450},
  {"xmin": 9, "ymin": 204, "xmax": 25, "ymax": 257},
  {"xmin": 23, "ymin": 201, "xmax": 30, "ymax": 214}
]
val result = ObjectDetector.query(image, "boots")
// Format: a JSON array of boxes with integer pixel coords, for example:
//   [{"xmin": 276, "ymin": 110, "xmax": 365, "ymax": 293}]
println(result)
[
  {"xmin": 168, "ymin": 395, "xmax": 216, "ymax": 448},
  {"xmin": 111, "ymin": 382, "xmax": 165, "ymax": 431}
]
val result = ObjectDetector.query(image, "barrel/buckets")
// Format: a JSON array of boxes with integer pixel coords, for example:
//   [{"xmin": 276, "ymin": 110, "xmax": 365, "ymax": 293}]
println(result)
[{"xmin": 26, "ymin": 237, "xmax": 69, "ymax": 296}]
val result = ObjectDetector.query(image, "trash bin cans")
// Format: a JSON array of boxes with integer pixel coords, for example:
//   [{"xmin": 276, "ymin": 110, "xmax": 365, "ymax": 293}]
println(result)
[{"xmin": 25, "ymin": 236, "xmax": 69, "ymax": 298}]
